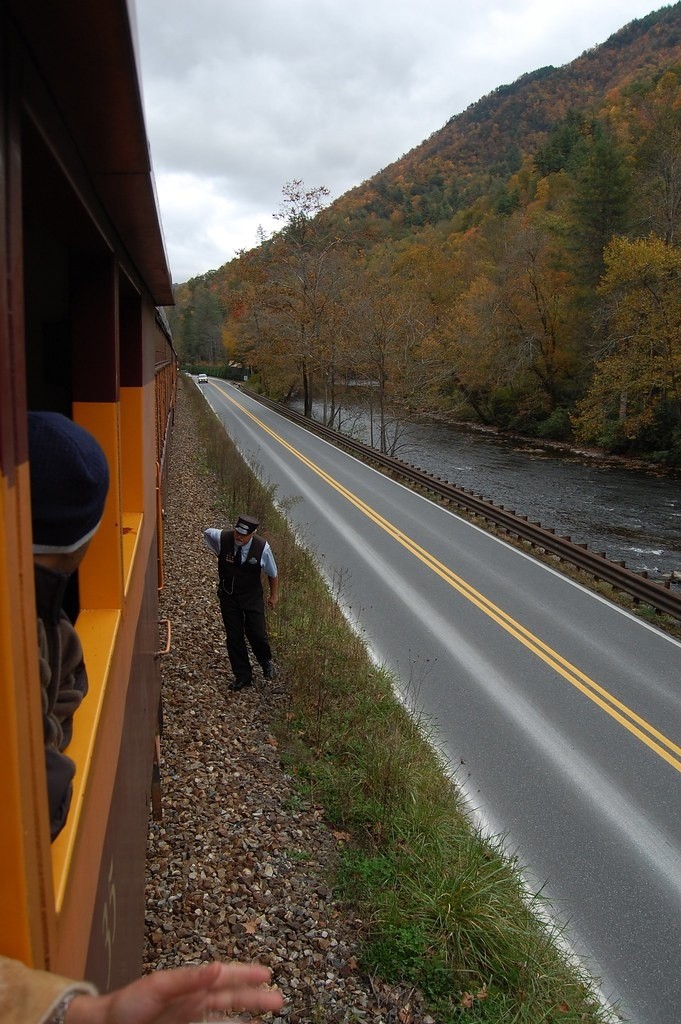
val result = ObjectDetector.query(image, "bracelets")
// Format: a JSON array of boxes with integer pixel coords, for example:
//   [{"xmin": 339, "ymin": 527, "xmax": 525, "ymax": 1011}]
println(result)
[{"xmin": 51, "ymin": 992, "xmax": 79, "ymax": 1024}]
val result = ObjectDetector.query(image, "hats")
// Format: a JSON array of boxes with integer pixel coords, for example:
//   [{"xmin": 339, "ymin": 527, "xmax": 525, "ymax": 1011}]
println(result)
[
  {"xmin": 27, "ymin": 413, "xmax": 109, "ymax": 553},
  {"xmin": 235, "ymin": 515, "xmax": 259, "ymax": 535}
]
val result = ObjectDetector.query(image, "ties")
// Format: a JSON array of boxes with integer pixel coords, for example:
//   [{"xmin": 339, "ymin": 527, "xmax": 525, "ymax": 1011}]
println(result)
[{"xmin": 235, "ymin": 546, "xmax": 242, "ymax": 565}]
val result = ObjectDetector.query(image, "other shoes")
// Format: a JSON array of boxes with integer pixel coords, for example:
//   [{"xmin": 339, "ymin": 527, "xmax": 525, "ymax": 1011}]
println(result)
[
  {"xmin": 261, "ymin": 661, "xmax": 274, "ymax": 680},
  {"xmin": 228, "ymin": 678, "xmax": 253, "ymax": 691}
]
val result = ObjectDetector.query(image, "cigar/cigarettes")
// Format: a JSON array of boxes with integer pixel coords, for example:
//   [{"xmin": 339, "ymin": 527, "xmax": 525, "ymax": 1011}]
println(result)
[{"xmin": 273, "ymin": 612, "xmax": 277, "ymax": 615}]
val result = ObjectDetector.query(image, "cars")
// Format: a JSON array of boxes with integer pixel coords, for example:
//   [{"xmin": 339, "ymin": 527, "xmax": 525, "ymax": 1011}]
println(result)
[{"xmin": 197, "ymin": 374, "xmax": 208, "ymax": 383}]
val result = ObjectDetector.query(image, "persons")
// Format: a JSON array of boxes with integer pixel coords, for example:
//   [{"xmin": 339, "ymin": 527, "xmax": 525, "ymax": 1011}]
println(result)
[
  {"xmin": 204, "ymin": 514, "xmax": 279, "ymax": 691},
  {"xmin": 0, "ymin": 956, "xmax": 283, "ymax": 1024},
  {"xmin": 28, "ymin": 411, "xmax": 111, "ymax": 844}
]
